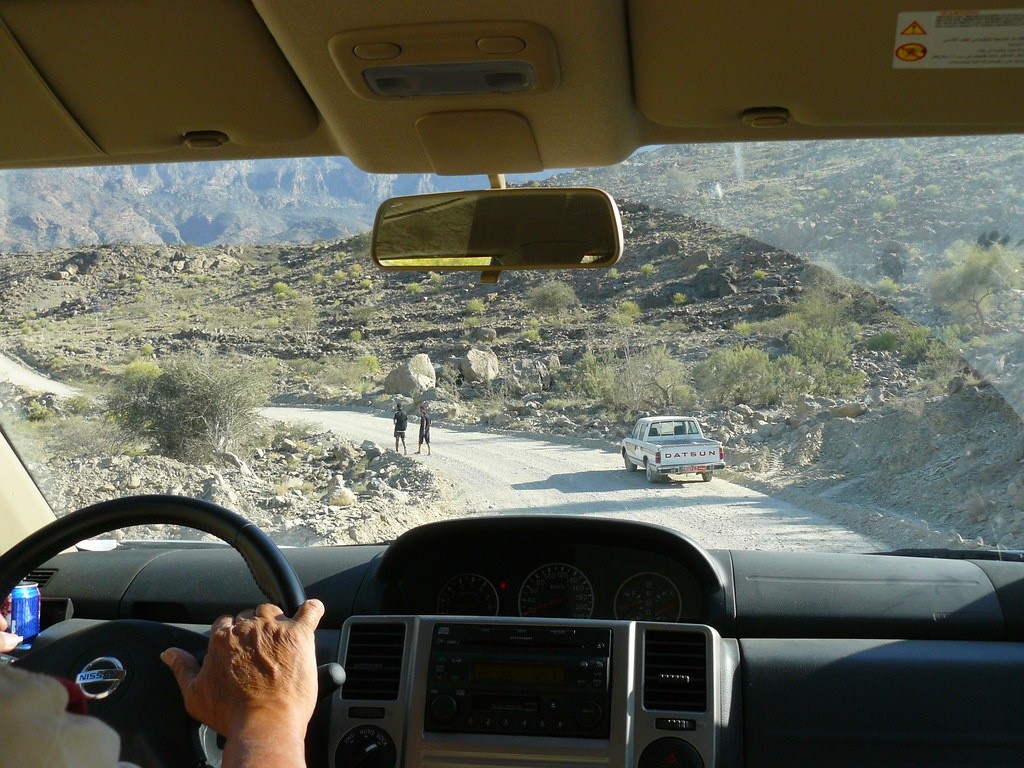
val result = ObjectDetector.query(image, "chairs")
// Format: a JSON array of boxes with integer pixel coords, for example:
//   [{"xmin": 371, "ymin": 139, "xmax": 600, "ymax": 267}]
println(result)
[
  {"xmin": 651, "ymin": 428, "xmax": 657, "ymax": 435},
  {"xmin": 674, "ymin": 426, "xmax": 685, "ymax": 435}
]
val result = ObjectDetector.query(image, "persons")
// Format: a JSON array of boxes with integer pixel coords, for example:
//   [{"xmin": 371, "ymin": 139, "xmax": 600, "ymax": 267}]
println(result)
[
  {"xmin": 415, "ymin": 406, "xmax": 430, "ymax": 455},
  {"xmin": 0, "ymin": 594, "xmax": 326, "ymax": 768},
  {"xmin": 393, "ymin": 403, "xmax": 408, "ymax": 455}
]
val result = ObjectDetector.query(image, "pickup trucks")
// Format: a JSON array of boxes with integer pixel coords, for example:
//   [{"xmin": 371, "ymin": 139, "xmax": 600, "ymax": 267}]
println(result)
[{"xmin": 621, "ymin": 415, "xmax": 726, "ymax": 484}]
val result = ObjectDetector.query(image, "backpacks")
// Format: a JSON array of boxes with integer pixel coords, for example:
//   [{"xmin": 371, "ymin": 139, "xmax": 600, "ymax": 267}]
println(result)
[{"xmin": 396, "ymin": 410, "xmax": 406, "ymax": 431}]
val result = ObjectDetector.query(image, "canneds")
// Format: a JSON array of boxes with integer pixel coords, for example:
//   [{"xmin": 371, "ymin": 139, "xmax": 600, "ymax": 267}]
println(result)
[{"xmin": 0, "ymin": 582, "xmax": 41, "ymax": 650}]
